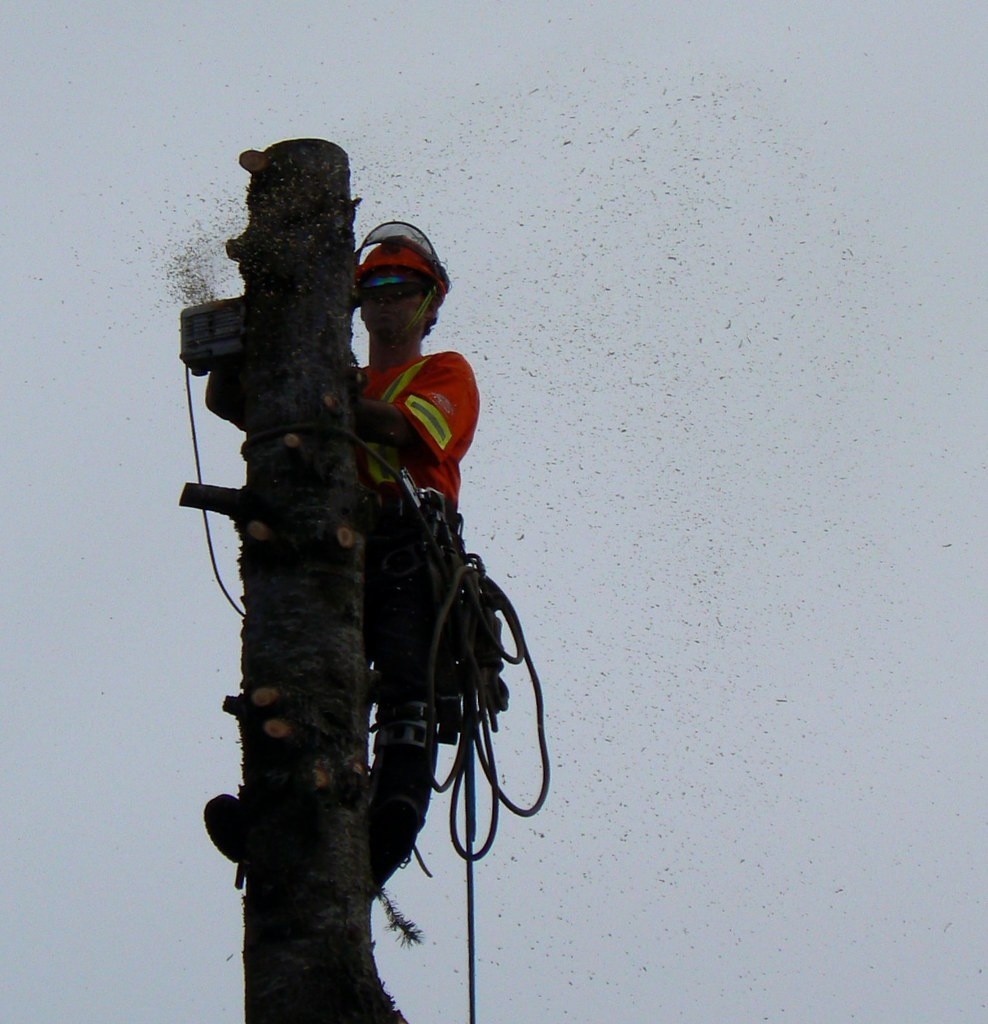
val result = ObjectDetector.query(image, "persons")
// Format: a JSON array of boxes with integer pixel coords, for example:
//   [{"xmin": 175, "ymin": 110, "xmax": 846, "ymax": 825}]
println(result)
[{"xmin": 205, "ymin": 220, "xmax": 481, "ymax": 905}]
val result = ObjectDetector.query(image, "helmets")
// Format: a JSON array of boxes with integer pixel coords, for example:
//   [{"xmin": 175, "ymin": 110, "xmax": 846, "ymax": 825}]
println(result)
[{"xmin": 355, "ymin": 222, "xmax": 448, "ymax": 296}]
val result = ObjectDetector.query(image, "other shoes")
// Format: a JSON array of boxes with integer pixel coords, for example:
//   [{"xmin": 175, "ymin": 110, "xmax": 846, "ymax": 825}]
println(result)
[
  {"xmin": 204, "ymin": 795, "xmax": 250, "ymax": 873},
  {"xmin": 368, "ymin": 795, "xmax": 420, "ymax": 897}
]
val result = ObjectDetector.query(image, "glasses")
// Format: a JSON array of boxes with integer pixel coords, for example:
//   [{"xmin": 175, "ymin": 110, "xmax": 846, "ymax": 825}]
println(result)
[{"xmin": 358, "ymin": 278, "xmax": 430, "ymax": 303}]
